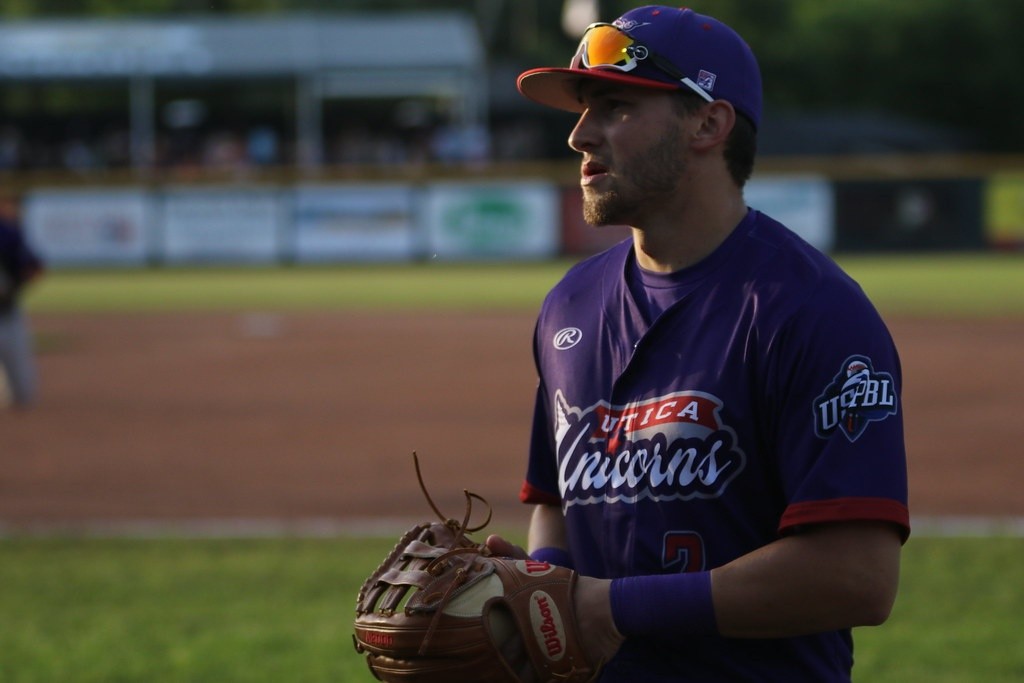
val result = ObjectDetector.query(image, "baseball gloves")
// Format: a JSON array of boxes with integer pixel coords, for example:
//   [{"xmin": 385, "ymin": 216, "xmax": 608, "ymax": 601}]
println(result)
[{"xmin": 350, "ymin": 517, "xmax": 596, "ymax": 683}]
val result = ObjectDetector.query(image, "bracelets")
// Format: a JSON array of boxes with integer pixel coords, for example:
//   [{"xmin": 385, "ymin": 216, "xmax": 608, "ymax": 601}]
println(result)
[
  {"xmin": 529, "ymin": 546, "xmax": 575, "ymax": 569},
  {"xmin": 609, "ymin": 570, "xmax": 721, "ymax": 637}
]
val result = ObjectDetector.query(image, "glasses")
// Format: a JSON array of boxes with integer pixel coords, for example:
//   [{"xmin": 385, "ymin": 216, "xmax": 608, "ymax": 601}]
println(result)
[{"xmin": 578, "ymin": 22, "xmax": 716, "ymax": 103}]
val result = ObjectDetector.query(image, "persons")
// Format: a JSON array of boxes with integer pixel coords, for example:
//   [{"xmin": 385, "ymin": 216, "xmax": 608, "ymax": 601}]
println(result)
[
  {"xmin": 352, "ymin": 4, "xmax": 910, "ymax": 682},
  {"xmin": 0, "ymin": 188, "xmax": 47, "ymax": 403},
  {"xmin": 0, "ymin": 103, "xmax": 492, "ymax": 175}
]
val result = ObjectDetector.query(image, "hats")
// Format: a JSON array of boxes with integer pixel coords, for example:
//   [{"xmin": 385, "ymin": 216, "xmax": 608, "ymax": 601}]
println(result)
[{"xmin": 516, "ymin": 5, "xmax": 763, "ymax": 131}]
